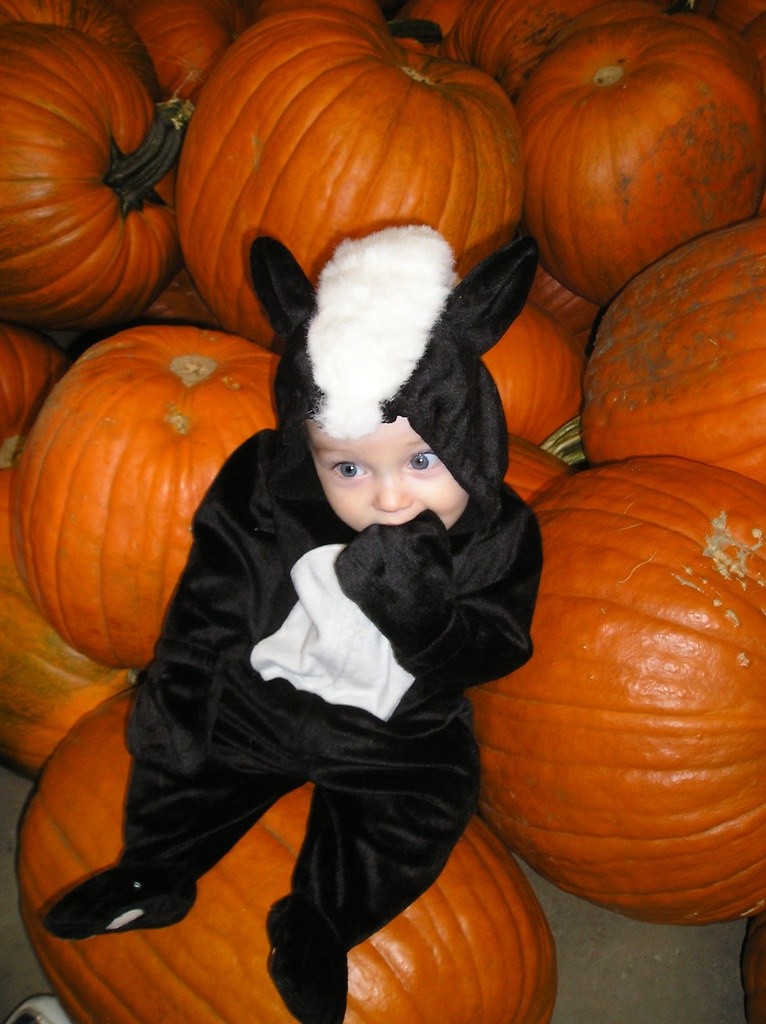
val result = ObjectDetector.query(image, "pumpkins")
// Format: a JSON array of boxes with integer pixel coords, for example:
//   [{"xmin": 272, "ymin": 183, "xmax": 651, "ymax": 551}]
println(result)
[{"xmin": 0, "ymin": 0, "xmax": 766, "ymax": 1024}]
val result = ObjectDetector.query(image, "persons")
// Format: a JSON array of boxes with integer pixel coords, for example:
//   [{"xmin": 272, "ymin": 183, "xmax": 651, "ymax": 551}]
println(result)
[{"xmin": 43, "ymin": 222, "xmax": 544, "ymax": 1024}]
